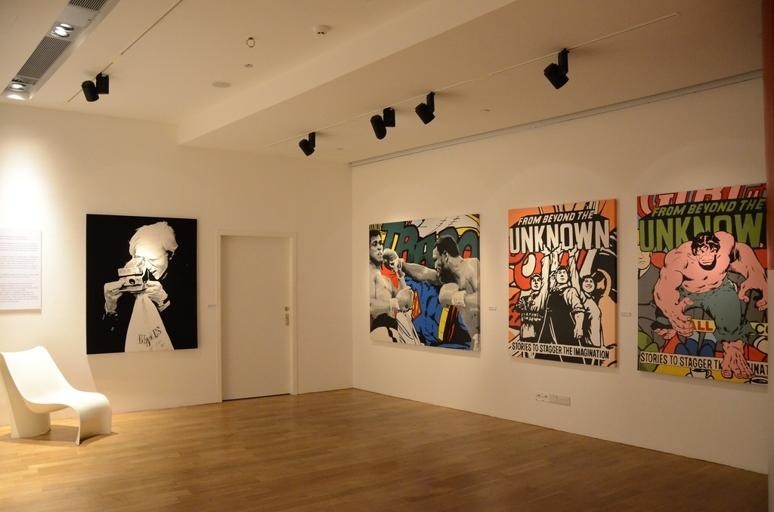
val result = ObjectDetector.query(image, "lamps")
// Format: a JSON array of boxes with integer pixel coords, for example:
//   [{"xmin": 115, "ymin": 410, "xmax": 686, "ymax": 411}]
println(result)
[
  {"xmin": 80, "ymin": 73, "xmax": 112, "ymax": 103},
  {"xmin": 298, "ymin": 132, "xmax": 316, "ymax": 156},
  {"xmin": 370, "ymin": 89, "xmax": 437, "ymax": 139},
  {"xmin": 543, "ymin": 47, "xmax": 571, "ymax": 90}
]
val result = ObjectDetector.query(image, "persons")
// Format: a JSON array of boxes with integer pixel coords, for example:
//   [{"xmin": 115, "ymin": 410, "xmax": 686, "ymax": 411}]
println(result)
[
  {"xmin": 514, "ymin": 272, "xmax": 543, "ymax": 358},
  {"xmin": 578, "ymin": 274, "xmax": 603, "ymax": 363},
  {"xmin": 87, "ymin": 220, "xmax": 194, "ymax": 352},
  {"xmin": 368, "ymin": 230, "xmax": 422, "ymax": 345},
  {"xmin": 653, "ymin": 229, "xmax": 768, "ymax": 378},
  {"xmin": 637, "ymin": 227, "xmax": 669, "ymax": 353},
  {"xmin": 532, "ymin": 261, "xmax": 586, "ymax": 359},
  {"xmin": 381, "ymin": 234, "xmax": 478, "ymax": 350}
]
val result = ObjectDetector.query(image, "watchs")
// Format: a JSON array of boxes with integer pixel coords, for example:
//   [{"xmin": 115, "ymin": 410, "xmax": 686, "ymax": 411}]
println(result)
[{"xmin": 153, "ymin": 295, "xmax": 169, "ymax": 308}]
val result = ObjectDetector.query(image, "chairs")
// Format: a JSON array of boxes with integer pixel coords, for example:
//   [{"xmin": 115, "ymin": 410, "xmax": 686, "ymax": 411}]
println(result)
[{"xmin": 0, "ymin": 346, "xmax": 112, "ymax": 446}]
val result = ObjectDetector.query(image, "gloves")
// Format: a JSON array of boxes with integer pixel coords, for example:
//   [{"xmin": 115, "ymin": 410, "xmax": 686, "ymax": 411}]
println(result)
[
  {"xmin": 439, "ymin": 283, "xmax": 467, "ymax": 307},
  {"xmin": 390, "ymin": 286, "xmax": 413, "ymax": 312},
  {"xmin": 383, "ymin": 248, "xmax": 406, "ymax": 271}
]
